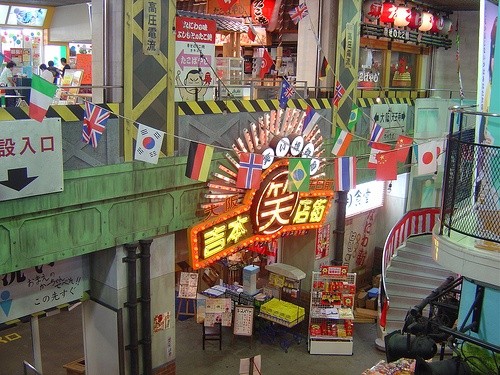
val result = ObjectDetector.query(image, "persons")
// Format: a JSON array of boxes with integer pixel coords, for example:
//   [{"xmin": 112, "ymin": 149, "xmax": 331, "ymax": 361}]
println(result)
[
  {"xmin": 0, "ymin": 61, "xmax": 16, "ymax": 87},
  {"xmin": 58, "ymin": 58, "xmax": 70, "ymax": 77},
  {"xmin": 48, "ymin": 60, "xmax": 59, "ymax": 83},
  {"xmin": 40, "ymin": 64, "xmax": 53, "ymax": 83}
]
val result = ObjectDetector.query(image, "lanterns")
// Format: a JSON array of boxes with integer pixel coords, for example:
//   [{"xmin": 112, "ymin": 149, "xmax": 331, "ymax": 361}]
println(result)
[{"xmin": 362, "ymin": 0, "xmax": 453, "ymax": 34}]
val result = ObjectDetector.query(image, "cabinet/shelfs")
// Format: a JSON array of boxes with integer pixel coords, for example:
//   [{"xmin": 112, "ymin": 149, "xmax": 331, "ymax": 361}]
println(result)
[{"xmin": 306, "ymin": 270, "xmax": 356, "ymax": 356}]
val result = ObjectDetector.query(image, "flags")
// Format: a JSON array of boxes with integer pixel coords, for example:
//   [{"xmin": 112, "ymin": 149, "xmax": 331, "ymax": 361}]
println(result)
[
  {"xmin": 367, "ymin": 122, "xmax": 386, "ymax": 146},
  {"xmin": 394, "ymin": 135, "xmax": 413, "ymax": 161},
  {"xmin": 331, "ymin": 126, "xmax": 353, "ymax": 157},
  {"xmin": 347, "ymin": 103, "xmax": 362, "ymax": 130},
  {"xmin": 185, "ymin": 141, "xmax": 214, "ymax": 182},
  {"xmin": 319, "ymin": 56, "xmax": 331, "ymax": 80},
  {"xmin": 436, "ymin": 141, "xmax": 444, "ymax": 165},
  {"xmin": 81, "ymin": 101, "xmax": 111, "ymax": 148},
  {"xmin": 288, "ymin": 158, "xmax": 311, "ymax": 192},
  {"xmin": 279, "ymin": 78, "xmax": 295, "ymax": 110},
  {"xmin": 418, "ymin": 142, "xmax": 438, "ymax": 174},
  {"xmin": 236, "ymin": 151, "xmax": 263, "ymax": 189},
  {"xmin": 334, "ymin": 80, "xmax": 346, "ymax": 107},
  {"xmin": 288, "ymin": 1, "xmax": 309, "ymax": 25},
  {"xmin": 367, "ymin": 142, "xmax": 391, "ymax": 170},
  {"xmin": 248, "ymin": 23, "xmax": 258, "ymax": 41},
  {"xmin": 302, "ymin": 105, "xmax": 322, "ymax": 136},
  {"xmin": 259, "ymin": 49, "xmax": 274, "ymax": 80},
  {"xmin": 134, "ymin": 123, "xmax": 164, "ymax": 165},
  {"xmin": 375, "ymin": 149, "xmax": 397, "ymax": 180},
  {"xmin": 334, "ymin": 156, "xmax": 356, "ymax": 191},
  {"xmin": 29, "ymin": 73, "xmax": 57, "ymax": 123}
]
